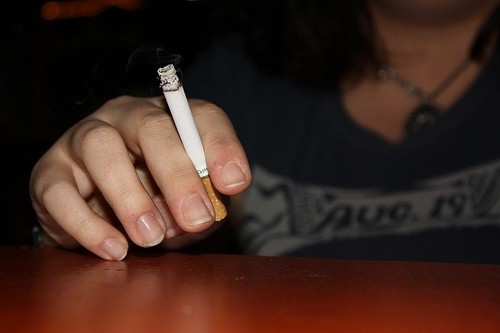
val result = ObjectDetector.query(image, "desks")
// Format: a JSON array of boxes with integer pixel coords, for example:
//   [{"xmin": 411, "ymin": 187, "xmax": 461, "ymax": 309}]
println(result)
[{"xmin": 0, "ymin": 245, "xmax": 500, "ymax": 333}]
